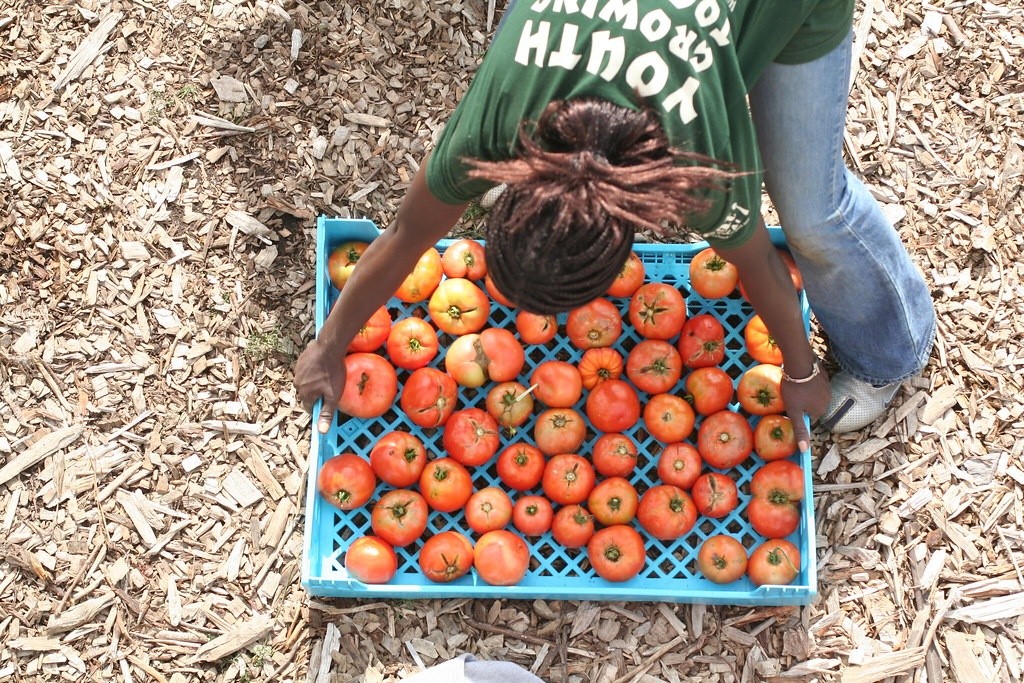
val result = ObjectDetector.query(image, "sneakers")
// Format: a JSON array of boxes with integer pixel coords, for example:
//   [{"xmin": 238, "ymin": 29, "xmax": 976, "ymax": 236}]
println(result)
[
  {"xmin": 820, "ymin": 367, "xmax": 902, "ymax": 434},
  {"xmin": 480, "ymin": 182, "xmax": 508, "ymax": 208}
]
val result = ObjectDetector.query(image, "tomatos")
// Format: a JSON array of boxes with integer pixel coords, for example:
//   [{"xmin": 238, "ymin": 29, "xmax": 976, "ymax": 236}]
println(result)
[{"xmin": 317, "ymin": 238, "xmax": 804, "ymax": 586}]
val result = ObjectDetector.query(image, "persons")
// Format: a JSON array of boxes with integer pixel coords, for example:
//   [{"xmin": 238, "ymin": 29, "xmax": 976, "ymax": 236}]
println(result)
[{"xmin": 295, "ymin": 0, "xmax": 940, "ymax": 451}]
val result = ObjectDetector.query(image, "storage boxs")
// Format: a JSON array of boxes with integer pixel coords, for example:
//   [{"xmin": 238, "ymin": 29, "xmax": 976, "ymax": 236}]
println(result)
[{"xmin": 300, "ymin": 214, "xmax": 819, "ymax": 608}]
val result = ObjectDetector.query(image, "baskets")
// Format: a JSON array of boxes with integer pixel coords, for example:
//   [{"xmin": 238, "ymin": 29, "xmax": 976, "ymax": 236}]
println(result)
[{"xmin": 301, "ymin": 217, "xmax": 819, "ymax": 607}]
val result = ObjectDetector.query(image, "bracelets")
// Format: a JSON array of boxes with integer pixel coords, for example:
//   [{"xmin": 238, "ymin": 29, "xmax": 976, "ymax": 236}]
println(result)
[{"xmin": 781, "ymin": 355, "xmax": 822, "ymax": 385}]
